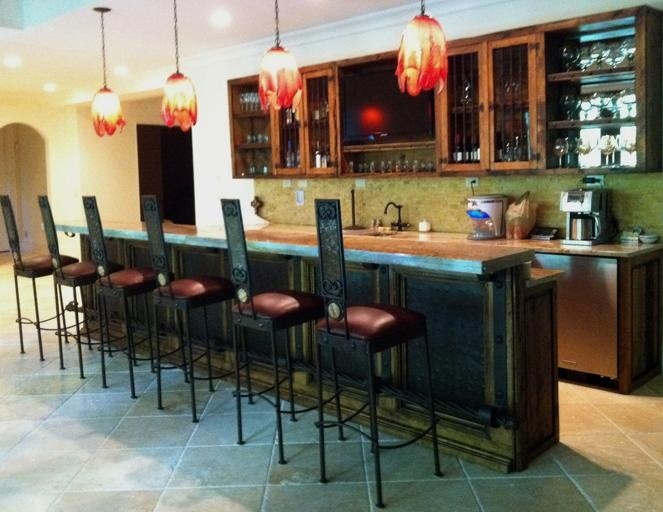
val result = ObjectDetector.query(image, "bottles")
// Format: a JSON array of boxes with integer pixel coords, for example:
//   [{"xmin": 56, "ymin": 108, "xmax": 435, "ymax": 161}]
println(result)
[
  {"xmin": 453, "ymin": 132, "xmax": 528, "ymax": 162},
  {"xmin": 239, "ymin": 92, "xmax": 260, "ymax": 112},
  {"xmin": 418, "ymin": 219, "xmax": 431, "ymax": 232},
  {"xmin": 285, "ymin": 92, "xmax": 329, "ymax": 168}
]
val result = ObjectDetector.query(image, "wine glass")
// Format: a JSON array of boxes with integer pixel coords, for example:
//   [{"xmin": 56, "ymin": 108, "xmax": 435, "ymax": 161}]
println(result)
[
  {"xmin": 561, "ymin": 89, "xmax": 637, "ymax": 120},
  {"xmin": 559, "ymin": 36, "xmax": 636, "ymax": 71},
  {"xmin": 554, "ymin": 135, "xmax": 639, "ymax": 168}
]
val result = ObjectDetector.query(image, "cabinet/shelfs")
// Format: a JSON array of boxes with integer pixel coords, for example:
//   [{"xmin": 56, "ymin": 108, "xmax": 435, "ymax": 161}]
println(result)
[
  {"xmin": 335, "ymin": 45, "xmax": 442, "ymax": 179},
  {"xmin": 529, "ymin": 253, "xmax": 621, "ymax": 381},
  {"xmin": 537, "ymin": 4, "xmax": 663, "ymax": 176},
  {"xmin": 441, "ymin": 26, "xmax": 539, "ymax": 178},
  {"xmin": 227, "ymin": 75, "xmax": 276, "ymax": 179},
  {"xmin": 274, "ymin": 60, "xmax": 337, "ymax": 179}
]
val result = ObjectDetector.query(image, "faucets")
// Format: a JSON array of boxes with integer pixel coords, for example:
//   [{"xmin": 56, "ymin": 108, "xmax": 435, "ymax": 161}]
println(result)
[{"xmin": 384, "ymin": 201, "xmax": 409, "ymax": 231}]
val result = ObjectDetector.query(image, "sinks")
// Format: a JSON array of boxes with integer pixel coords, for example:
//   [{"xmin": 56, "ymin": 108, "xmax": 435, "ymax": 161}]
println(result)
[{"xmin": 344, "ymin": 228, "xmax": 400, "ymax": 237}]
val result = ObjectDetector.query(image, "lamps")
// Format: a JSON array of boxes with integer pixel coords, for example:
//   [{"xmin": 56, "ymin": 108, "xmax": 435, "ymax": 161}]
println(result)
[
  {"xmin": 91, "ymin": 7, "xmax": 126, "ymax": 138},
  {"xmin": 160, "ymin": 0, "xmax": 197, "ymax": 133},
  {"xmin": 394, "ymin": 0, "xmax": 449, "ymax": 97},
  {"xmin": 258, "ymin": 0, "xmax": 303, "ymax": 112}
]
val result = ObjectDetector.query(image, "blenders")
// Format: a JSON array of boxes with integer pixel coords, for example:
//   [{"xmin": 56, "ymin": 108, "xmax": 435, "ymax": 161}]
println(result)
[{"xmin": 466, "ymin": 194, "xmax": 507, "ymax": 241}]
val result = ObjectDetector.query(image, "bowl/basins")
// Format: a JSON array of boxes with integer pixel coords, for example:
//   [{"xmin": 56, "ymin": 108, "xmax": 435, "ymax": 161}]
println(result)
[{"xmin": 638, "ymin": 234, "xmax": 658, "ymax": 244}]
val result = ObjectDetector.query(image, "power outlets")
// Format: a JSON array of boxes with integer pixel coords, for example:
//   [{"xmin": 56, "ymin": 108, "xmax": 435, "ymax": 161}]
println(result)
[{"xmin": 465, "ymin": 178, "xmax": 479, "ymax": 188}]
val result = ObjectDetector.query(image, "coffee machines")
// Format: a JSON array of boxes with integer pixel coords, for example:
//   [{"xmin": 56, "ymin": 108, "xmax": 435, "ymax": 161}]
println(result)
[{"xmin": 559, "ymin": 186, "xmax": 619, "ymax": 247}]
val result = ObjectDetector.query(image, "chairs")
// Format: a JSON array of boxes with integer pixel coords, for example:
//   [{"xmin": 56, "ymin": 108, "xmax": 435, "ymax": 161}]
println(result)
[
  {"xmin": 141, "ymin": 194, "xmax": 255, "ymax": 422},
  {"xmin": 38, "ymin": 195, "xmax": 125, "ymax": 379},
  {"xmin": 220, "ymin": 198, "xmax": 347, "ymax": 464},
  {"xmin": 82, "ymin": 195, "xmax": 175, "ymax": 399},
  {"xmin": 0, "ymin": 194, "xmax": 93, "ymax": 362},
  {"xmin": 312, "ymin": 197, "xmax": 444, "ymax": 508}
]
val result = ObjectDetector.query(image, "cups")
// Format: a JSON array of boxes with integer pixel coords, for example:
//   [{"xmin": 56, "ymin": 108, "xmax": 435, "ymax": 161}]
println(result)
[
  {"xmin": 348, "ymin": 160, "xmax": 434, "ymax": 173},
  {"xmin": 245, "ymin": 133, "xmax": 270, "ymax": 144}
]
